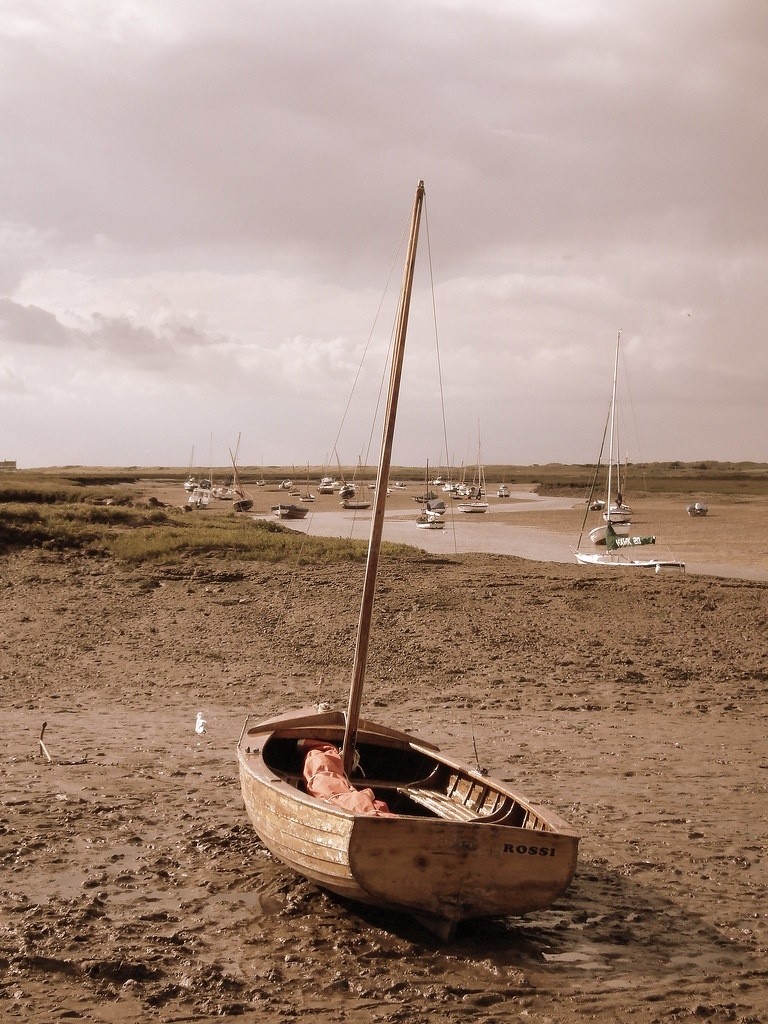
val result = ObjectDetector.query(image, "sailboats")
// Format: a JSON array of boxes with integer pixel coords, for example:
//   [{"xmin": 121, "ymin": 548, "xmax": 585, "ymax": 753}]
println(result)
[
  {"xmin": 570, "ymin": 393, "xmax": 686, "ymax": 573},
  {"xmin": 229, "ymin": 449, "xmax": 254, "ymax": 512},
  {"xmin": 333, "ymin": 446, "xmax": 355, "ymax": 498},
  {"xmin": 288, "ymin": 459, "xmax": 341, "ymax": 502},
  {"xmin": 184, "ymin": 430, "xmax": 242, "ymax": 500},
  {"xmin": 429, "ymin": 450, "xmax": 464, "ymax": 499},
  {"xmin": 588, "ymin": 329, "xmax": 635, "ymax": 548},
  {"xmin": 341, "ymin": 456, "xmax": 373, "ymax": 510},
  {"xmin": 257, "ymin": 453, "xmax": 266, "ymax": 486},
  {"xmin": 238, "ymin": 178, "xmax": 584, "ymax": 923},
  {"xmin": 458, "ymin": 418, "xmax": 488, "ymax": 513},
  {"xmin": 411, "ymin": 458, "xmax": 447, "ymax": 529}
]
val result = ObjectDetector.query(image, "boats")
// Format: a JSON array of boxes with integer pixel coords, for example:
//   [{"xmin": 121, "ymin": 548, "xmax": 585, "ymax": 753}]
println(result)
[
  {"xmin": 497, "ymin": 484, "xmax": 511, "ymax": 498},
  {"xmin": 279, "ymin": 478, "xmax": 291, "ymax": 489},
  {"xmin": 272, "ymin": 504, "xmax": 309, "ymax": 519},
  {"xmin": 188, "ymin": 488, "xmax": 210, "ymax": 510},
  {"xmin": 687, "ymin": 501, "xmax": 708, "ymax": 517},
  {"xmin": 367, "ymin": 482, "xmax": 407, "ymax": 495}
]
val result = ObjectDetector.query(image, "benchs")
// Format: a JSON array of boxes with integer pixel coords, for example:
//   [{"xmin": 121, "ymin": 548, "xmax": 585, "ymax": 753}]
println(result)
[
  {"xmin": 395, "ymin": 762, "xmax": 511, "ymax": 824},
  {"xmin": 277, "ymin": 766, "xmax": 409, "ymax": 794}
]
